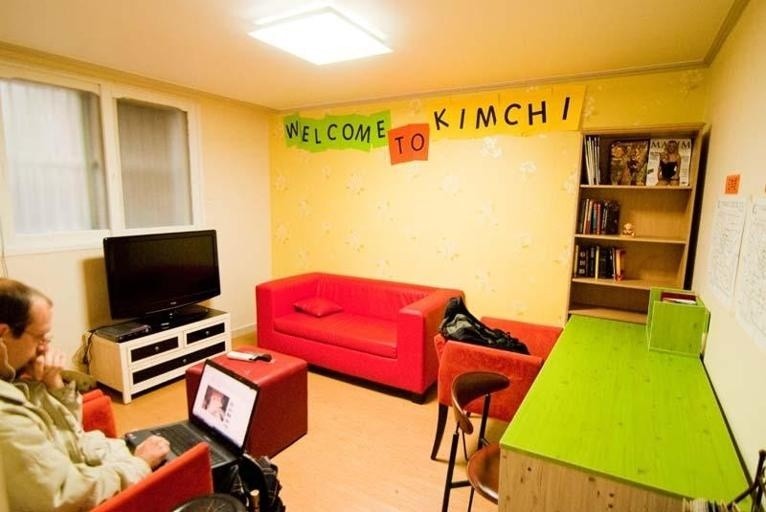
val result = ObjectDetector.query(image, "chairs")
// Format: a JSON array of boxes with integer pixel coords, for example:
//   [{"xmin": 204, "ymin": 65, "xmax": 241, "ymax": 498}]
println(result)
[
  {"xmin": 78, "ymin": 389, "xmax": 102, "ymax": 402},
  {"xmin": 431, "ymin": 316, "xmax": 563, "ymax": 458},
  {"xmin": 84, "ymin": 396, "xmax": 213, "ymax": 512},
  {"xmin": 441, "ymin": 371, "xmax": 511, "ymax": 512}
]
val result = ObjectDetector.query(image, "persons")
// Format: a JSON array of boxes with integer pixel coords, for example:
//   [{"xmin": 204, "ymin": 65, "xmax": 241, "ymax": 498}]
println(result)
[{"xmin": 1, "ymin": 277, "xmax": 170, "ymax": 512}]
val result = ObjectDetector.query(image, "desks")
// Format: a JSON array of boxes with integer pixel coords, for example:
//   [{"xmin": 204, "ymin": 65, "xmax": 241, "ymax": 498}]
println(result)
[{"xmin": 499, "ymin": 313, "xmax": 757, "ymax": 511}]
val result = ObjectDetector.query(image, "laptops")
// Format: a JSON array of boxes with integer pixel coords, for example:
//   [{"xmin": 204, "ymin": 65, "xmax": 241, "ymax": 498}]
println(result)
[{"xmin": 125, "ymin": 359, "xmax": 260, "ymax": 472}]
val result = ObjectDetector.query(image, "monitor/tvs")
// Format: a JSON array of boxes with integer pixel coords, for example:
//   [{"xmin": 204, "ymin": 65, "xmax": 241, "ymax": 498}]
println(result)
[{"xmin": 103, "ymin": 230, "xmax": 220, "ymax": 328}]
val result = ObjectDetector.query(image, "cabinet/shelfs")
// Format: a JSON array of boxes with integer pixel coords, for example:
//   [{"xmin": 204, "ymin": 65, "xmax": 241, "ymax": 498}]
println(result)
[
  {"xmin": 565, "ymin": 119, "xmax": 707, "ymax": 326},
  {"xmin": 83, "ymin": 309, "xmax": 232, "ymax": 404}
]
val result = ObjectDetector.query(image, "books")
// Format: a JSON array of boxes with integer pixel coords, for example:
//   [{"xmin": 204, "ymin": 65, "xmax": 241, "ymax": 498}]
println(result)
[{"xmin": 574, "ymin": 135, "xmax": 692, "ymax": 282}]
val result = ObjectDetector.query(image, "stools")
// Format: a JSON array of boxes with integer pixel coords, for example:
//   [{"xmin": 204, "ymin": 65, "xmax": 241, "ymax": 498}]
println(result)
[{"xmin": 186, "ymin": 344, "xmax": 307, "ymax": 461}]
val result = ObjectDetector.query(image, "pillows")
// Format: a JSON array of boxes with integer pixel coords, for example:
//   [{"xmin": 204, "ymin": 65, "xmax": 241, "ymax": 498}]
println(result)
[{"xmin": 295, "ymin": 297, "xmax": 342, "ymax": 317}]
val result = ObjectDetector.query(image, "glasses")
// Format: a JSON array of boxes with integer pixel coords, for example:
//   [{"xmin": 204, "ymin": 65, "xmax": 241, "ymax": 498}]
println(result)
[{"xmin": 25, "ymin": 329, "xmax": 54, "ymax": 346}]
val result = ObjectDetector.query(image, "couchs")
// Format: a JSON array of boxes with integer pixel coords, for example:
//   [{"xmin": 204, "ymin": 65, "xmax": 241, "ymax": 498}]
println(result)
[{"xmin": 255, "ymin": 270, "xmax": 465, "ymax": 406}]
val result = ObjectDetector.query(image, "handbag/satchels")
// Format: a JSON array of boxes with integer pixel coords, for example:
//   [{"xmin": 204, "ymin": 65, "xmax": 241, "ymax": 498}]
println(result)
[{"xmin": 437, "ymin": 296, "xmax": 529, "ymax": 355}]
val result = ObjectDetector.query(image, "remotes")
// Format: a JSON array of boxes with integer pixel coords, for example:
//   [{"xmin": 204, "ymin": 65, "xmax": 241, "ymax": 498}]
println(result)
[
  {"xmin": 227, "ymin": 351, "xmax": 258, "ymax": 362},
  {"xmin": 242, "ymin": 351, "xmax": 272, "ymax": 362}
]
